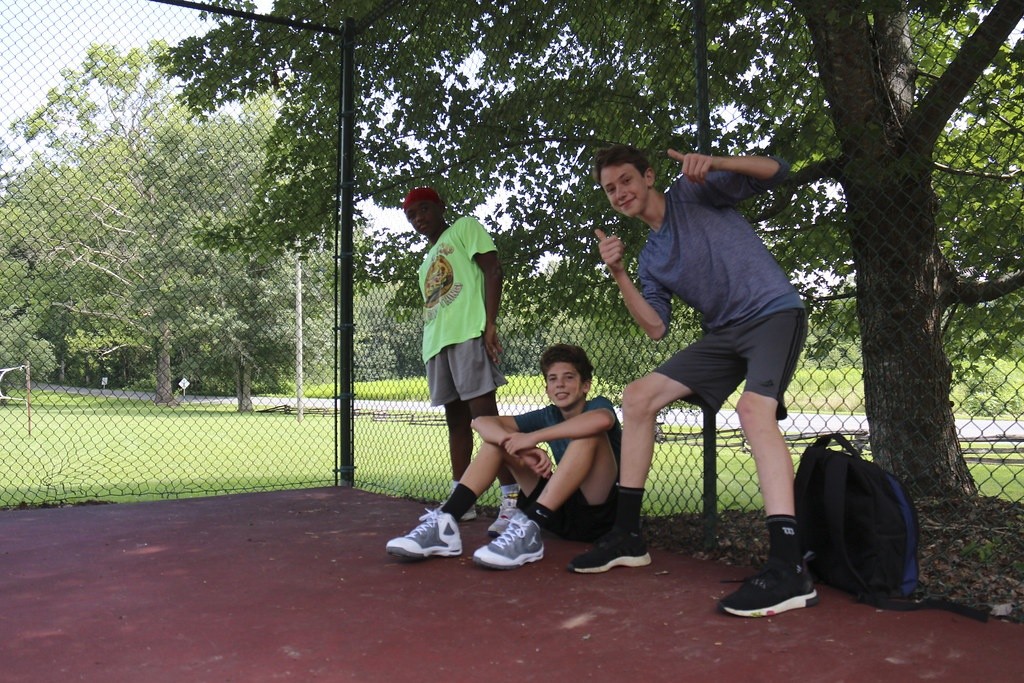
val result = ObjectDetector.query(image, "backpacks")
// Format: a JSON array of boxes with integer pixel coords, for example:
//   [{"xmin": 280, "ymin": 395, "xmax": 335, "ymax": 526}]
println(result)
[{"xmin": 794, "ymin": 432, "xmax": 926, "ymax": 610}]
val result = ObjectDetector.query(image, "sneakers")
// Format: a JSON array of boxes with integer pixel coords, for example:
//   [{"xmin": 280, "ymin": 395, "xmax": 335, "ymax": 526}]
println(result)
[
  {"xmin": 719, "ymin": 564, "xmax": 818, "ymax": 618},
  {"xmin": 487, "ymin": 498, "xmax": 518, "ymax": 537},
  {"xmin": 566, "ymin": 529, "xmax": 653, "ymax": 573},
  {"xmin": 386, "ymin": 511, "xmax": 463, "ymax": 558},
  {"xmin": 440, "ymin": 490, "xmax": 476, "ymax": 521},
  {"xmin": 473, "ymin": 509, "xmax": 544, "ymax": 570}
]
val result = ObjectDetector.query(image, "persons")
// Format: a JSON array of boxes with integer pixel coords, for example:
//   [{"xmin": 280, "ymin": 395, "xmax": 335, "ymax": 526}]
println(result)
[
  {"xmin": 380, "ymin": 342, "xmax": 623, "ymax": 570},
  {"xmin": 404, "ymin": 185, "xmax": 523, "ymax": 538},
  {"xmin": 568, "ymin": 147, "xmax": 817, "ymax": 615}
]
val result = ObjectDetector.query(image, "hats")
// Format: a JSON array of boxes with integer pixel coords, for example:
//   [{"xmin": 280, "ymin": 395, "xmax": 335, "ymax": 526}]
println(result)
[{"xmin": 403, "ymin": 187, "xmax": 446, "ymax": 214}]
